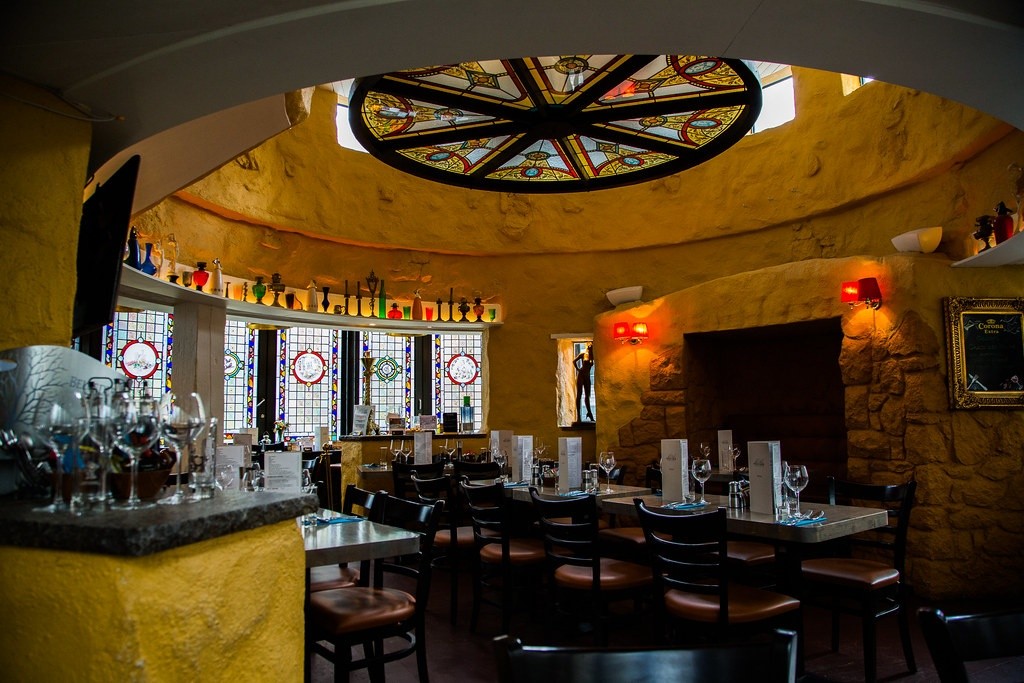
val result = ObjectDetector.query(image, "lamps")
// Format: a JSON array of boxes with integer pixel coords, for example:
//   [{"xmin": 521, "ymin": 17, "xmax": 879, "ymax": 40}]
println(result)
[
  {"xmin": 613, "ymin": 322, "xmax": 648, "ymax": 345},
  {"xmin": 839, "ymin": 276, "xmax": 883, "ymax": 310}
]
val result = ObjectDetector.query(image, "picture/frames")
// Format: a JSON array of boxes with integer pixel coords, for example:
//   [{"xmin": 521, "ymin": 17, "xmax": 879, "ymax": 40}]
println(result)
[{"xmin": 942, "ymin": 296, "xmax": 1024, "ymax": 407}]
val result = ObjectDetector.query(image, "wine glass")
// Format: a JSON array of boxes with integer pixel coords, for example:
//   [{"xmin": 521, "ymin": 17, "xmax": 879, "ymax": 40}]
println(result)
[
  {"xmin": 781, "ymin": 460, "xmax": 810, "ymax": 519},
  {"xmin": 400, "ymin": 440, "xmax": 413, "ymax": 464},
  {"xmin": 389, "ymin": 439, "xmax": 402, "ymax": 463},
  {"xmin": 29, "ymin": 385, "xmax": 208, "ymax": 514},
  {"xmin": 599, "ymin": 452, "xmax": 617, "ymax": 493},
  {"xmin": 692, "ymin": 460, "xmax": 713, "ymax": 505},
  {"xmin": 492, "ymin": 449, "xmax": 510, "ymax": 484},
  {"xmin": 488, "ymin": 438, "xmax": 499, "ymax": 463},
  {"xmin": 535, "ymin": 437, "xmax": 545, "ymax": 461},
  {"xmin": 444, "ymin": 438, "xmax": 457, "ymax": 467},
  {"xmin": 699, "ymin": 442, "xmax": 741, "ymax": 472}
]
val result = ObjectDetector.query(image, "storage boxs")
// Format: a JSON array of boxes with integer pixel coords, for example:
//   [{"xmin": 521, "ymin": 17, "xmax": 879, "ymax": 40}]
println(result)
[
  {"xmin": 746, "ymin": 441, "xmax": 782, "ymax": 513},
  {"xmin": 414, "ymin": 432, "xmax": 434, "ymax": 464},
  {"xmin": 717, "ymin": 429, "xmax": 733, "ymax": 470},
  {"xmin": 512, "ymin": 435, "xmax": 533, "ymax": 482},
  {"xmin": 659, "ymin": 439, "xmax": 691, "ymax": 501},
  {"xmin": 556, "ymin": 437, "xmax": 584, "ymax": 489},
  {"xmin": 491, "ymin": 430, "xmax": 514, "ymax": 467}
]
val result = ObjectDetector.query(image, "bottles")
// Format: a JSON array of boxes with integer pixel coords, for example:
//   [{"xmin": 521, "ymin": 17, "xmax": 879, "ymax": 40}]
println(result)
[
  {"xmin": 582, "ymin": 470, "xmax": 591, "ymax": 492},
  {"xmin": 728, "ymin": 480, "xmax": 750, "ymax": 509}
]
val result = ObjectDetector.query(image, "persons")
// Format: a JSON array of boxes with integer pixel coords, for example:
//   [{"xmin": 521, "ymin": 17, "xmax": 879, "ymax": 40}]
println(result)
[{"xmin": 573, "ymin": 341, "xmax": 594, "ymax": 421}]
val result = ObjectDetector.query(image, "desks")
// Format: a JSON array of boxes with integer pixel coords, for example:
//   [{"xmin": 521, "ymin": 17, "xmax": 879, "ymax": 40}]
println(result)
[
  {"xmin": 467, "ymin": 478, "xmax": 513, "ymax": 496},
  {"xmin": 598, "ymin": 493, "xmax": 741, "ymax": 650},
  {"xmin": 511, "ymin": 483, "xmax": 652, "ymax": 605},
  {"xmin": 356, "ymin": 465, "xmax": 466, "ymax": 500},
  {"xmin": 297, "ymin": 507, "xmax": 419, "ymax": 683},
  {"xmin": 692, "ymin": 493, "xmax": 888, "ymax": 683}
]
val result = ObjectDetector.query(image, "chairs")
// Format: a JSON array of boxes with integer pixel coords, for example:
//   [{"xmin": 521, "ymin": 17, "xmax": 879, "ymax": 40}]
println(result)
[{"xmin": 305, "ymin": 450, "xmax": 1024, "ymax": 683}]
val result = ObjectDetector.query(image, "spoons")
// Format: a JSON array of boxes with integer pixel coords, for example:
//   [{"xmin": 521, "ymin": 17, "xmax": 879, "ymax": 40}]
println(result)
[
  {"xmin": 780, "ymin": 511, "xmax": 825, "ymax": 524},
  {"xmin": 725, "ymin": 466, "xmax": 746, "ymax": 472}
]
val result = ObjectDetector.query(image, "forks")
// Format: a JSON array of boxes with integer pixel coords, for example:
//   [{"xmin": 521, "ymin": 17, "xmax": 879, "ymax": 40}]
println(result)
[{"xmin": 787, "ymin": 509, "xmax": 812, "ymax": 526}]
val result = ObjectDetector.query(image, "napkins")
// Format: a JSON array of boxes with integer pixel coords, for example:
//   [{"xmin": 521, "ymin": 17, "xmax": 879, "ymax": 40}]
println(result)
[
  {"xmin": 309, "ymin": 514, "xmax": 366, "ymax": 525},
  {"xmin": 559, "ymin": 490, "xmax": 589, "ymax": 497},
  {"xmin": 503, "ymin": 481, "xmax": 532, "ymax": 488},
  {"xmin": 770, "ymin": 517, "xmax": 826, "ymax": 526},
  {"xmin": 659, "ymin": 500, "xmax": 710, "ymax": 511}
]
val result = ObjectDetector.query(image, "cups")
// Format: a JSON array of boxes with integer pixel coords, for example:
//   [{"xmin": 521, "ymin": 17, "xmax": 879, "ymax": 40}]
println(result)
[
  {"xmin": 300, "ymin": 486, "xmax": 319, "ymax": 528},
  {"xmin": 214, "ymin": 463, "xmax": 313, "ymax": 492},
  {"xmin": 590, "ymin": 463, "xmax": 601, "ymax": 493},
  {"xmin": 184, "ymin": 418, "xmax": 219, "ymax": 500},
  {"xmin": 774, "ymin": 477, "xmax": 791, "ymax": 523},
  {"xmin": 379, "ymin": 446, "xmax": 393, "ymax": 468},
  {"xmin": 684, "ymin": 457, "xmax": 699, "ymax": 505}
]
